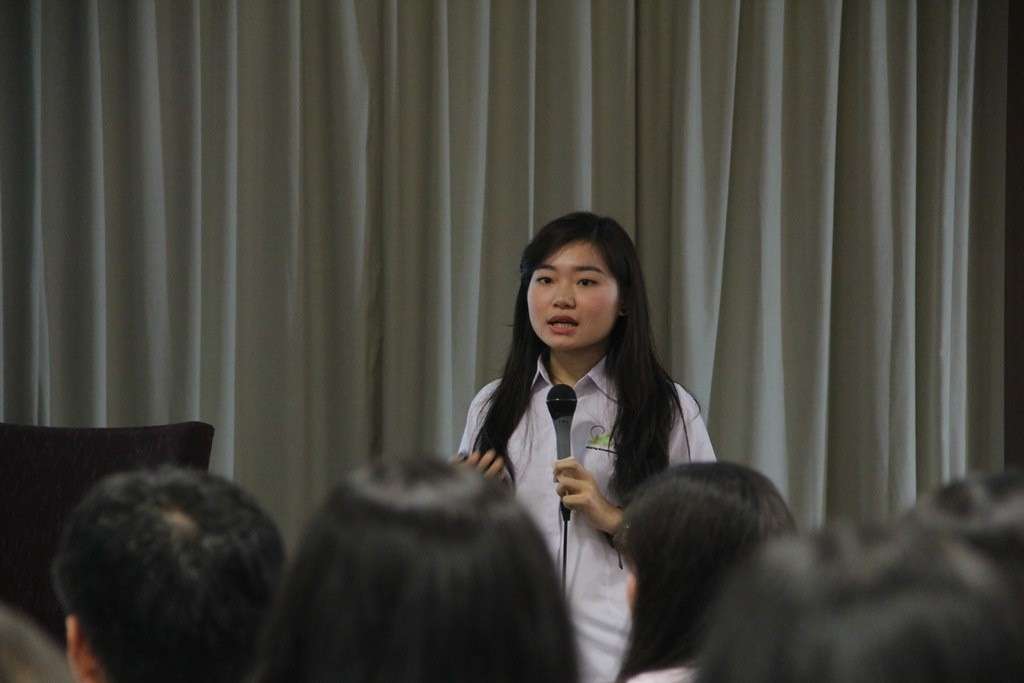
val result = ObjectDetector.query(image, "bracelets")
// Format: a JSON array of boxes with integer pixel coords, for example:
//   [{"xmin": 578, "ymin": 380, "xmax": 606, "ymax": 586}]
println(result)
[{"xmin": 606, "ymin": 506, "xmax": 631, "ymax": 569}]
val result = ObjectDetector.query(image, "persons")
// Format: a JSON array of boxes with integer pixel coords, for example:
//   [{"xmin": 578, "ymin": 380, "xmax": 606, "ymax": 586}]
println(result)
[
  {"xmin": 447, "ymin": 211, "xmax": 716, "ymax": 683},
  {"xmin": 0, "ymin": 454, "xmax": 1024, "ymax": 683}
]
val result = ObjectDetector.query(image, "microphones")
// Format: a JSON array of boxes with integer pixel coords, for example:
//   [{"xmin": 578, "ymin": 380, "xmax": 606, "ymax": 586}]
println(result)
[{"xmin": 545, "ymin": 384, "xmax": 578, "ymax": 520}]
